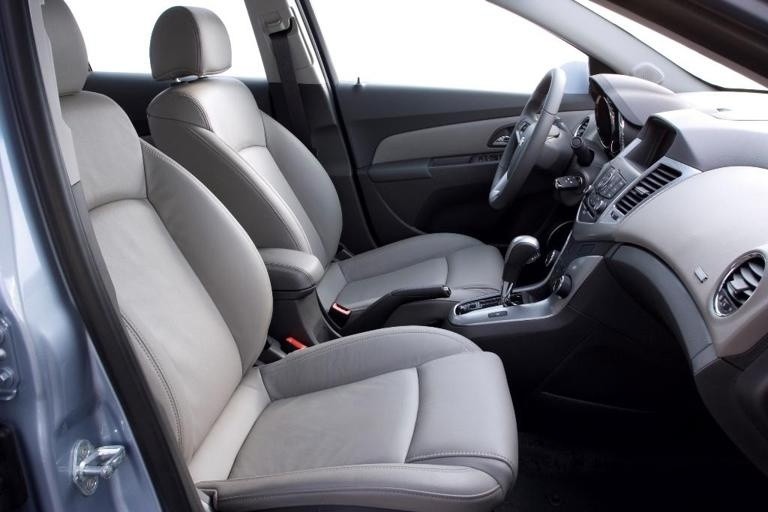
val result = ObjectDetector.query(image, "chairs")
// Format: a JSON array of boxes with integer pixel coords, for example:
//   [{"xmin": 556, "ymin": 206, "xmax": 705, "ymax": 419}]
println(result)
[
  {"xmin": 147, "ymin": 3, "xmax": 511, "ymax": 335},
  {"xmin": 39, "ymin": 1, "xmax": 529, "ymax": 510}
]
